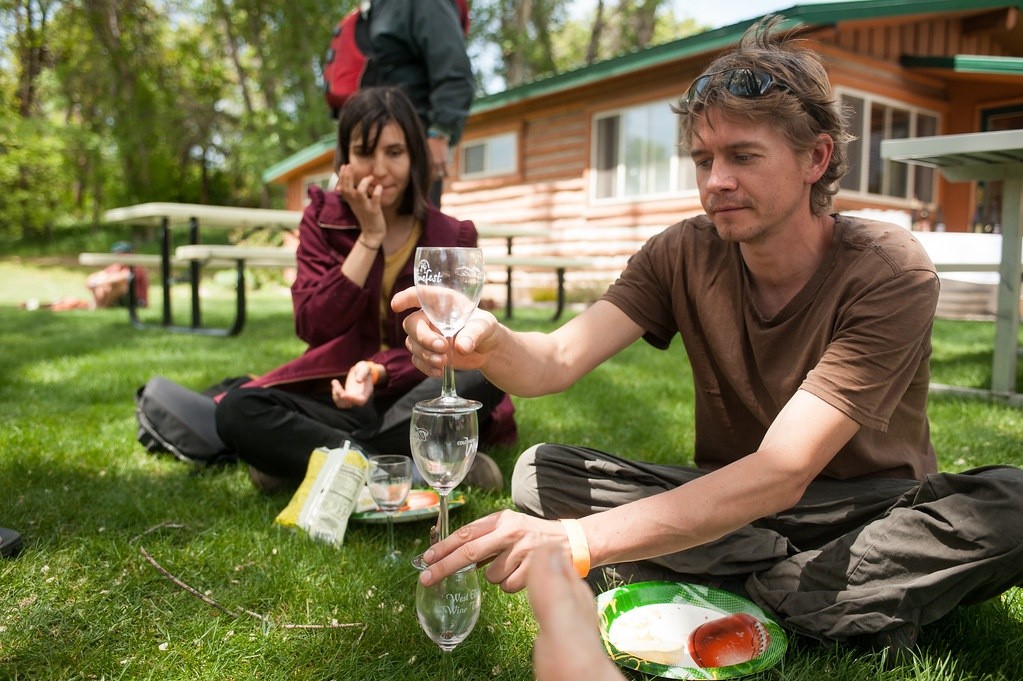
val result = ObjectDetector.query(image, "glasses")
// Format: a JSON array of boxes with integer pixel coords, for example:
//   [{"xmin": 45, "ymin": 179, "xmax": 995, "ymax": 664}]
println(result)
[{"xmin": 685, "ymin": 66, "xmax": 826, "ymax": 128}]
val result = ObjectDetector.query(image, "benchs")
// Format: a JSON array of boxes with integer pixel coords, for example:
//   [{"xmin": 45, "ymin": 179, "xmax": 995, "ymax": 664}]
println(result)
[
  {"xmin": 175, "ymin": 244, "xmax": 594, "ymax": 338},
  {"xmin": 78, "ymin": 253, "xmax": 298, "ymax": 338}
]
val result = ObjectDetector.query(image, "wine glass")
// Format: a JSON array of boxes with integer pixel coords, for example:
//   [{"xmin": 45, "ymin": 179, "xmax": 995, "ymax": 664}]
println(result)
[
  {"xmin": 416, "ymin": 568, "xmax": 481, "ymax": 681},
  {"xmin": 414, "ymin": 247, "xmax": 485, "ymax": 411},
  {"xmin": 366, "ymin": 455, "xmax": 412, "ymax": 568},
  {"xmin": 409, "ymin": 409, "xmax": 479, "ymax": 574}
]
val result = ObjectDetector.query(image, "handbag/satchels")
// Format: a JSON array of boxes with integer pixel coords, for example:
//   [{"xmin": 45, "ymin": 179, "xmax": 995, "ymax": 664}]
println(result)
[{"xmin": 135, "ymin": 376, "xmax": 225, "ymax": 467}]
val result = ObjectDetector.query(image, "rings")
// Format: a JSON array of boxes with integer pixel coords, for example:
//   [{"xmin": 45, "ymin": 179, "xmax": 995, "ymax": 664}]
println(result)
[{"xmin": 437, "ymin": 170, "xmax": 445, "ymax": 176}]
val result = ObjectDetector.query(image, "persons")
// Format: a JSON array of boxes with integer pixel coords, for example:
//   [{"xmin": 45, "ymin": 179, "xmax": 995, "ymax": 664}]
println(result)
[
  {"xmin": 361, "ymin": 0, "xmax": 476, "ymax": 212},
  {"xmin": 214, "ymin": 86, "xmax": 519, "ymax": 496},
  {"xmin": 86, "ymin": 241, "xmax": 148, "ymax": 308},
  {"xmin": 526, "ymin": 542, "xmax": 629, "ymax": 681},
  {"xmin": 390, "ymin": 12, "xmax": 1023, "ymax": 637}
]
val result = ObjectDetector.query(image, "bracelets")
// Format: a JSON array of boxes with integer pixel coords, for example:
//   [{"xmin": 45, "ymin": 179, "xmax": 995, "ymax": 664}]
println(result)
[
  {"xmin": 427, "ymin": 127, "xmax": 450, "ymax": 143},
  {"xmin": 358, "ymin": 240, "xmax": 379, "ymax": 251},
  {"xmin": 367, "ymin": 361, "xmax": 379, "ymax": 384},
  {"xmin": 557, "ymin": 517, "xmax": 591, "ymax": 578}
]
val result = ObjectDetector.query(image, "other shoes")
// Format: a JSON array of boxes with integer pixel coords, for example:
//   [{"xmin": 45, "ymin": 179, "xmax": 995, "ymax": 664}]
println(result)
[
  {"xmin": 886, "ymin": 623, "xmax": 919, "ymax": 655},
  {"xmin": 605, "ymin": 563, "xmax": 725, "ymax": 588}
]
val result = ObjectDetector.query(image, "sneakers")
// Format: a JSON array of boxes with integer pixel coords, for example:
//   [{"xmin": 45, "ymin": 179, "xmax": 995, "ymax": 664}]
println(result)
[
  {"xmin": 459, "ymin": 452, "xmax": 504, "ymax": 493},
  {"xmin": 249, "ymin": 465, "xmax": 279, "ymax": 496}
]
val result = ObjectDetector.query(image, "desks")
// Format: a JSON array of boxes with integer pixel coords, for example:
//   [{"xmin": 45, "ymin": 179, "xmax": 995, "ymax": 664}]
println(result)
[{"xmin": 95, "ymin": 202, "xmax": 550, "ymax": 327}]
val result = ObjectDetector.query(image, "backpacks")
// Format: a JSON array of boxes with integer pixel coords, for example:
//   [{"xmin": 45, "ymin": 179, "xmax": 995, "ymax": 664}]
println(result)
[{"xmin": 323, "ymin": 5, "xmax": 395, "ymax": 110}]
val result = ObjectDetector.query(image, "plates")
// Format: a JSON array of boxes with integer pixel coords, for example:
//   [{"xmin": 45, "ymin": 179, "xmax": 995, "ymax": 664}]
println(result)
[
  {"xmin": 595, "ymin": 581, "xmax": 787, "ymax": 680},
  {"xmin": 347, "ymin": 486, "xmax": 469, "ymax": 523}
]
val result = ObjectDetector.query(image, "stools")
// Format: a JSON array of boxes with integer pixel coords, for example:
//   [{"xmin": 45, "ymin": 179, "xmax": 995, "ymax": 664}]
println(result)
[{"xmin": 881, "ymin": 131, "xmax": 1023, "ymax": 408}]
